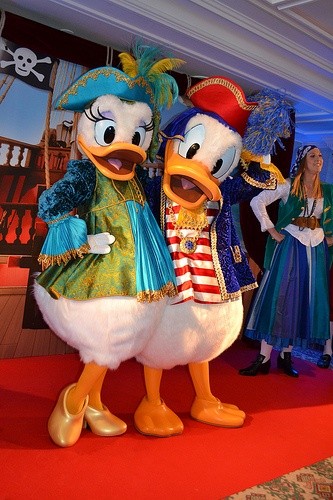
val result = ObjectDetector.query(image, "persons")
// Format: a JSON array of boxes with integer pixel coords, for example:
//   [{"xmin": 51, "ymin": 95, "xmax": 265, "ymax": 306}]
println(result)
[{"xmin": 237, "ymin": 144, "xmax": 332, "ymax": 377}]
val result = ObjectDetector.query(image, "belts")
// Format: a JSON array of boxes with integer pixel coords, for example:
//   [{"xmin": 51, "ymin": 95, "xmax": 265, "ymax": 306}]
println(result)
[{"xmin": 290, "ymin": 216, "xmax": 321, "ymax": 230}]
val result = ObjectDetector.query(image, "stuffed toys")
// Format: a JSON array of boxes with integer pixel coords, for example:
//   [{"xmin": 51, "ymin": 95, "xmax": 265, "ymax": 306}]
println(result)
[{"xmin": 33, "ymin": 94, "xmax": 285, "ymax": 446}]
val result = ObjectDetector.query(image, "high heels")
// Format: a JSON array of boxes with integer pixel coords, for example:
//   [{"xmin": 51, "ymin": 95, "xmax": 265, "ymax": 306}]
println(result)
[
  {"xmin": 239, "ymin": 353, "xmax": 271, "ymax": 376},
  {"xmin": 277, "ymin": 352, "xmax": 299, "ymax": 377}
]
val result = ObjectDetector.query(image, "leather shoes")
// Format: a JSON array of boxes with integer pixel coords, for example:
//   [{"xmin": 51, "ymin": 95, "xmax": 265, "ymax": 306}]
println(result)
[{"xmin": 317, "ymin": 354, "xmax": 332, "ymax": 368}]
